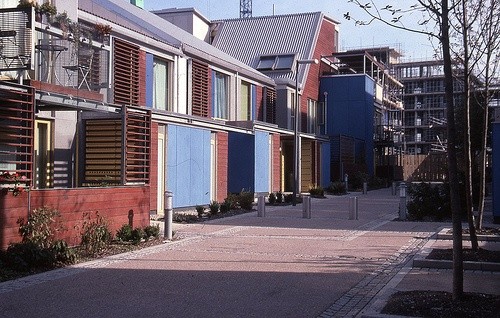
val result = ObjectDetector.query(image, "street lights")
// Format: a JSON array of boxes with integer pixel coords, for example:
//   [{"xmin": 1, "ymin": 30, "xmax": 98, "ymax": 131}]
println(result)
[{"xmin": 292, "ymin": 59, "xmax": 319, "ymax": 207}]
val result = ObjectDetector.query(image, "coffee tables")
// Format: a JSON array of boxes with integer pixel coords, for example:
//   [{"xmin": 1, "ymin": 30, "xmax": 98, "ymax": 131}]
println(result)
[{"xmin": 34, "ymin": 45, "xmax": 68, "ymax": 87}]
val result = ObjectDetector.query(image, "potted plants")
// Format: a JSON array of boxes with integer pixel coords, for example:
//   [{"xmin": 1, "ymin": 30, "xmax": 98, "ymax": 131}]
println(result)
[{"xmin": 18, "ymin": 0, "xmax": 114, "ymax": 46}]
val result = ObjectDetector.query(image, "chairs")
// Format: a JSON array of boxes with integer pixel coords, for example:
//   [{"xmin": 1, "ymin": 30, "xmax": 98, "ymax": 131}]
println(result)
[
  {"xmin": 62, "ymin": 49, "xmax": 96, "ymax": 91},
  {"xmin": 0, "ymin": 30, "xmax": 32, "ymax": 85}
]
